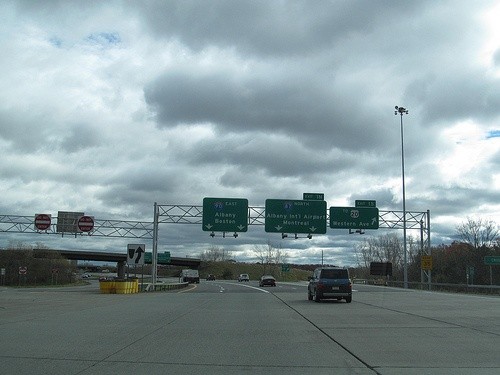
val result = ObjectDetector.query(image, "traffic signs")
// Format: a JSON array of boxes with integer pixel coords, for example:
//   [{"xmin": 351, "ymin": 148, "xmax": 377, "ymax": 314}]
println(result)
[
  {"xmin": 202, "ymin": 197, "xmax": 248, "ymax": 232},
  {"xmin": 329, "ymin": 206, "xmax": 380, "ymax": 230},
  {"xmin": 264, "ymin": 198, "xmax": 327, "ymax": 235}
]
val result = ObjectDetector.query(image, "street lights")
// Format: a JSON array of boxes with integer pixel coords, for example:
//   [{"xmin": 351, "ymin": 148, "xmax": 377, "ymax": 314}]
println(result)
[{"xmin": 394, "ymin": 104, "xmax": 409, "ymax": 289}]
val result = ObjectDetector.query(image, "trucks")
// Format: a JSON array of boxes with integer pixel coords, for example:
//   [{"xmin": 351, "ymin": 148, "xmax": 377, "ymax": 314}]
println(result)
[{"xmin": 181, "ymin": 270, "xmax": 200, "ymax": 284}]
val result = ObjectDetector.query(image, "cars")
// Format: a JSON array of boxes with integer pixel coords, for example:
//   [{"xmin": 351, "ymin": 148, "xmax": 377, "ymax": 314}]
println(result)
[
  {"xmin": 259, "ymin": 275, "xmax": 277, "ymax": 287},
  {"xmin": 238, "ymin": 273, "xmax": 251, "ymax": 282},
  {"xmin": 206, "ymin": 274, "xmax": 216, "ymax": 281}
]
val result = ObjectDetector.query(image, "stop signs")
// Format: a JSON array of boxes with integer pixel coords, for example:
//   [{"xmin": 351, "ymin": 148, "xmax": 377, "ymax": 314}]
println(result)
[
  {"xmin": 35, "ymin": 214, "xmax": 51, "ymax": 230},
  {"xmin": 77, "ymin": 216, "xmax": 94, "ymax": 232}
]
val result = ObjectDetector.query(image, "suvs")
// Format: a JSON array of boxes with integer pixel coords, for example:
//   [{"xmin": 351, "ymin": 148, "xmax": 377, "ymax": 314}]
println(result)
[{"xmin": 307, "ymin": 266, "xmax": 353, "ymax": 303}]
